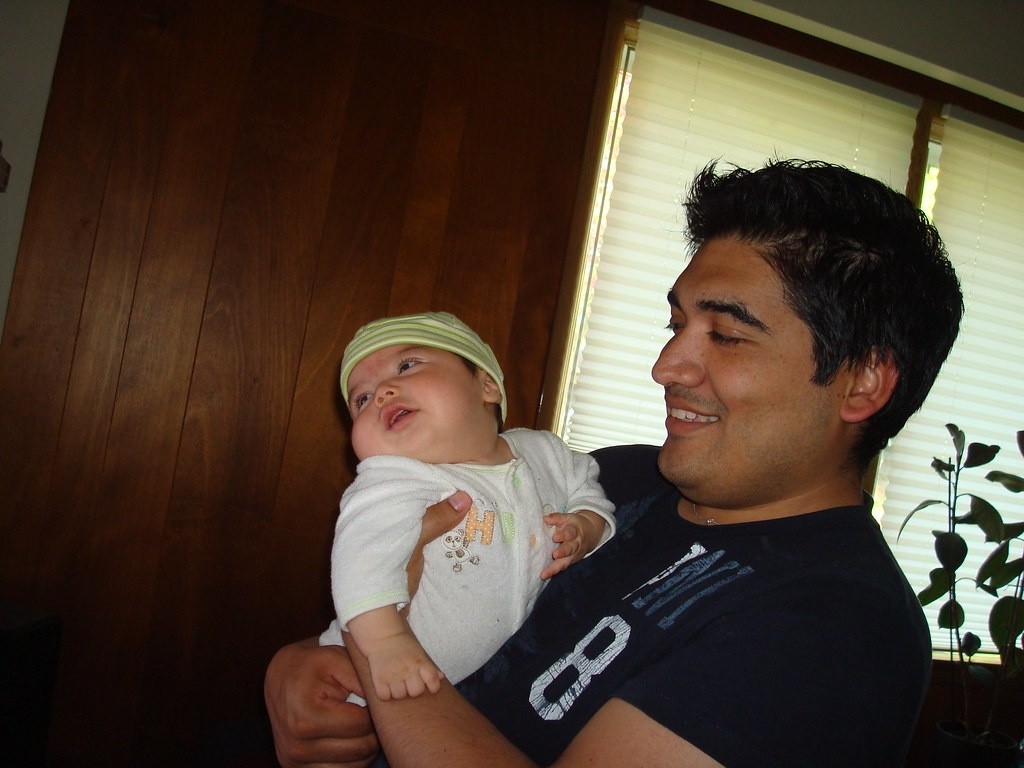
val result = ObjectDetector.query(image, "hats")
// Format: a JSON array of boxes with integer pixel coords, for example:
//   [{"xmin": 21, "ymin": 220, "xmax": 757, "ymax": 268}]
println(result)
[{"xmin": 339, "ymin": 312, "xmax": 506, "ymax": 425}]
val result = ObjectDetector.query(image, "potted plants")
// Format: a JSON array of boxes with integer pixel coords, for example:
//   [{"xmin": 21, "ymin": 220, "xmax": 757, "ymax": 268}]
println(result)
[{"xmin": 920, "ymin": 422, "xmax": 1024, "ymax": 768}]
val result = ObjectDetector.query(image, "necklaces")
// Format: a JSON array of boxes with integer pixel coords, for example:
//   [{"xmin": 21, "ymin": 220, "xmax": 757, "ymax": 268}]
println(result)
[{"xmin": 693, "ymin": 503, "xmax": 719, "ymax": 526}]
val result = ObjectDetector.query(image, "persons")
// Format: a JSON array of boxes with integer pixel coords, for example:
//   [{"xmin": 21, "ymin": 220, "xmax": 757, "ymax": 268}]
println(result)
[
  {"xmin": 263, "ymin": 157, "xmax": 964, "ymax": 768},
  {"xmin": 328, "ymin": 311, "xmax": 617, "ymax": 703}
]
